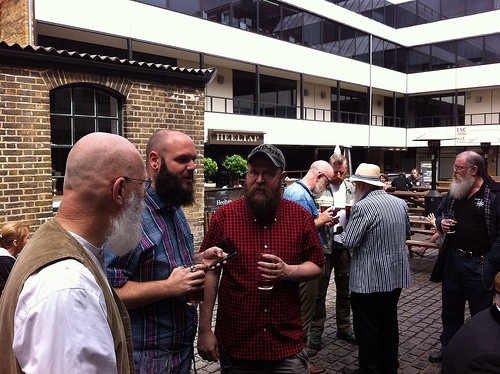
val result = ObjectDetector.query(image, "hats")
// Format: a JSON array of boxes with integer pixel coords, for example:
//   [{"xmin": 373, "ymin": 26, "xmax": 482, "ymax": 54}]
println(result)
[
  {"xmin": 346, "ymin": 162, "xmax": 386, "ymax": 186},
  {"xmin": 246, "ymin": 144, "xmax": 286, "ymax": 171}
]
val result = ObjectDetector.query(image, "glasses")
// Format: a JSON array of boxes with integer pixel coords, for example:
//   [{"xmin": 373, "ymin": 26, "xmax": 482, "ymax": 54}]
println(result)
[
  {"xmin": 110, "ymin": 176, "xmax": 153, "ymax": 191},
  {"xmin": 319, "ymin": 172, "xmax": 332, "ymax": 186},
  {"xmin": 451, "ymin": 166, "xmax": 473, "ymax": 172}
]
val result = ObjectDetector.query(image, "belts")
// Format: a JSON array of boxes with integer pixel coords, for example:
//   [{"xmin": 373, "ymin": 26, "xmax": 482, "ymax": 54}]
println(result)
[{"xmin": 449, "ymin": 242, "xmax": 487, "ymax": 257}]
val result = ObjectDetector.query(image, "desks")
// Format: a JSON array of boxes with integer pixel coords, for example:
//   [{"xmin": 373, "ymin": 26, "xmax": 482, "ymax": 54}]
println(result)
[
  {"xmin": 388, "ymin": 189, "xmax": 425, "ymax": 198},
  {"xmin": 408, "ymin": 186, "xmax": 450, "ymax": 194}
]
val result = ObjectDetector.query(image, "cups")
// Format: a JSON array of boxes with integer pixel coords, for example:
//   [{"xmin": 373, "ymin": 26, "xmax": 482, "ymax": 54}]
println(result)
[
  {"xmin": 320, "ymin": 204, "xmax": 330, "ymax": 214},
  {"xmin": 256, "ymin": 253, "xmax": 274, "ymax": 290},
  {"xmin": 345, "ymin": 205, "xmax": 351, "ymax": 221},
  {"xmin": 442, "ymin": 211, "xmax": 456, "ymax": 234}
]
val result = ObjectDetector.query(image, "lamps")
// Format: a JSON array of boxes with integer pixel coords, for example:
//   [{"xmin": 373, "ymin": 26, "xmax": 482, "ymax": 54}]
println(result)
[
  {"xmin": 304, "ymin": 89, "xmax": 310, "ymax": 96},
  {"xmin": 217, "ymin": 75, "xmax": 225, "ymax": 84},
  {"xmin": 377, "ymin": 100, "xmax": 381, "ymax": 106},
  {"xmin": 476, "ymin": 96, "xmax": 481, "ymax": 103},
  {"xmin": 321, "ymin": 91, "xmax": 327, "ymax": 98}
]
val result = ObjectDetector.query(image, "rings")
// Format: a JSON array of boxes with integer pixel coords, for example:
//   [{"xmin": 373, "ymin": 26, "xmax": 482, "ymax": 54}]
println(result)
[
  {"xmin": 190, "ymin": 264, "xmax": 196, "ymax": 272},
  {"xmin": 275, "ymin": 264, "xmax": 277, "ymax": 269}
]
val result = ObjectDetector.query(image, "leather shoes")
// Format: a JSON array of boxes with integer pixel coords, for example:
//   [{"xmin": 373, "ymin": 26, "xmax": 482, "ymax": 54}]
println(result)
[
  {"xmin": 428, "ymin": 350, "xmax": 441, "ymax": 363},
  {"xmin": 336, "ymin": 331, "xmax": 358, "ymax": 345},
  {"xmin": 309, "ymin": 335, "xmax": 321, "ymax": 348},
  {"xmin": 308, "ymin": 361, "xmax": 326, "ymax": 374},
  {"xmin": 305, "ymin": 348, "xmax": 317, "ymax": 357}
]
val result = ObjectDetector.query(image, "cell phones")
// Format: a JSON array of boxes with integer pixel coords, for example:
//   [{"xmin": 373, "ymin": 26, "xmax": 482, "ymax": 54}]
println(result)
[{"xmin": 209, "ymin": 251, "xmax": 238, "ymax": 268}]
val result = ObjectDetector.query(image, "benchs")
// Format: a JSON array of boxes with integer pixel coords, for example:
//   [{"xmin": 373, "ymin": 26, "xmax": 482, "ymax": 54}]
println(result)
[
  {"xmin": 409, "ymin": 206, "xmax": 425, "ymax": 212},
  {"xmin": 408, "ymin": 215, "xmax": 433, "ymax": 229},
  {"xmin": 404, "ymin": 238, "xmax": 442, "ymax": 258},
  {"xmin": 409, "ymin": 224, "xmax": 437, "ymax": 236}
]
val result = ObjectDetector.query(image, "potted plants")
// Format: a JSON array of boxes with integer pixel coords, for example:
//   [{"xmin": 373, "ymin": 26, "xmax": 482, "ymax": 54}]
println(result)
[
  {"xmin": 204, "ymin": 156, "xmax": 219, "ymax": 183},
  {"xmin": 223, "ymin": 154, "xmax": 249, "ymax": 187}
]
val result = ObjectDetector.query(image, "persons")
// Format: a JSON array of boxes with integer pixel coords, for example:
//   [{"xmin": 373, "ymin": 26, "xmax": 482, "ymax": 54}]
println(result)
[
  {"xmin": 428, "ymin": 150, "xmax": 500, "ymax": 363},
  {"xmin": 306, "ymin": 152, "xmax": 357, "ymax": 357},
  {"xmin": 379, "ymin": 173, "xmax": 393, "ymax": 191},
  {"xmin": 391, "ymin": 171, "xmax": 413, "ymax": 192},
  {"xmin": 412, "ymin": 210, "xmax": 443, "ymax": 257},
  {"xmin": 340, "ymin": 153, "xmax": 410, "ymax": 374},
  {"xmin": 0, "ymin": 130, "xmax": 326, "ymax": 374},
  {"xmin": 440, "ymin": 272, "xmax": 500, "ymax": 374},
  {"xmin": 407, "ymin": 168, "xmax": 426, "ymax": 187},
  {"xmin": 271, "ymin": 160, "xmax": 340, "ymax": 363}
]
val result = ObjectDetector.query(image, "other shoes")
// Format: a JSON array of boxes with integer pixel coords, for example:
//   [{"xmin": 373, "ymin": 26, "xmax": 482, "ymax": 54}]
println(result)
[{"xmin": 342, "ymin": 366, "xmax": 359, "ymax": 374}]
